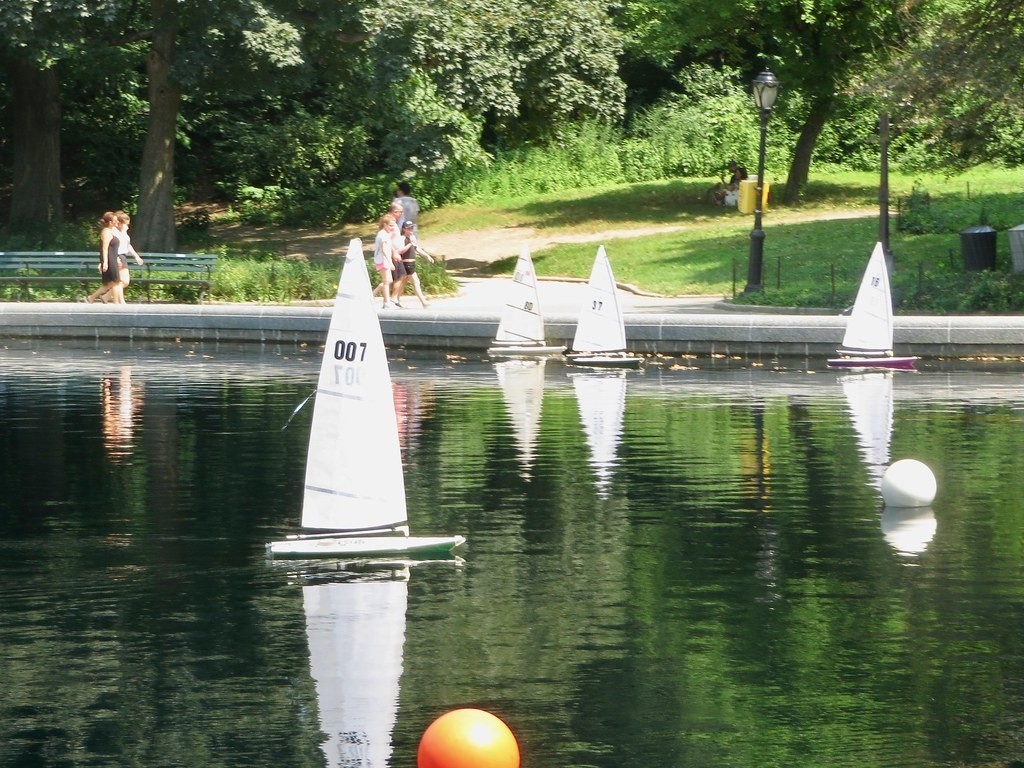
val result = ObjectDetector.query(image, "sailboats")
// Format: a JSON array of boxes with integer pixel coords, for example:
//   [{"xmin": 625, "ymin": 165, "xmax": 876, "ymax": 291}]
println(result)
[
  {"xmin": 826, "ymin": 365, "xmax": 918, "ymax": 489},
  {"xmin": 270, "ymin": 551, "xmax": 467, "ymax": 768},
  {"xmin": 566, "ymin": 367, "xmax": 645, "ymax": 497},
  {"xmin": 567, "ymin": 245, "xmax": 645, "ymax": 367},
  {"xmin": 265, "ymin": 238, "xmax": 466, "ymax": 560},
  {"xmin": 490, "ymin": 353, "xmax": 567, "ymax": 483},
  {"xmin": 487, "ymin": 243, "xmax": 568, "ymax": 354},
  {"xmin": 827, "ymin": 242, "xmax": 922, "ymax": 366}
]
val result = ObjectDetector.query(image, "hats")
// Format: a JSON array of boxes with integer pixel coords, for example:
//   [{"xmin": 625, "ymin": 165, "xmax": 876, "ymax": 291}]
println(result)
[{"xmin": 403, "ymin": 221, "xmax": 414, "ymax": 229}]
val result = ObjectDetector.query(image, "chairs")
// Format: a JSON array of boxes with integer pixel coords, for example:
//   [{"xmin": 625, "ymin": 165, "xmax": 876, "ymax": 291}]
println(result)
[{"xmin": 725, "ymin": 174, "xmax": 758, "ymax": 206}]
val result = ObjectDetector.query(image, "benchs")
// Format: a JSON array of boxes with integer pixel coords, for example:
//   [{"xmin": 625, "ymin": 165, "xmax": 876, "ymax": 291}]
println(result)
[{"xmin": 0, "ymin": 252, "xmax": 218, "ymax": 304}]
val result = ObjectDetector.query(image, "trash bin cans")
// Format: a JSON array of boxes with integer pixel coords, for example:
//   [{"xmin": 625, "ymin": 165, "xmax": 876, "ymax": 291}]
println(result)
[
  {"xmin": 959, "ymin": 226, "xmax": 998, "ymax": 271},
  {"xmin": 1007, "ymin": 224, "xmax": 1024, "ymax": 276}
]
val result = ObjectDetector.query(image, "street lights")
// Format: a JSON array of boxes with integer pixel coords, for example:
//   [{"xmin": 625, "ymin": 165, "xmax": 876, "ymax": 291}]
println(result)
[{"xmin": 745, "ymin": 68, "xmax": 781, "ymax": 292}]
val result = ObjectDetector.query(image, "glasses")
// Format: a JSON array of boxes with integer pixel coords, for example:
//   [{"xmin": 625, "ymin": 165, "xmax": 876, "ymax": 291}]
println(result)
[{"xmin": 112, "ymin": 220, "xmax": 118, "ymax": 222}]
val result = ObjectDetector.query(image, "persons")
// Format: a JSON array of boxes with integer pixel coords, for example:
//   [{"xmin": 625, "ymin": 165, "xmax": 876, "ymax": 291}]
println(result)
[
  {"xmin": 373, "ymin": 181, "xmax": 434, "ymax": 309},
  {"xmin": 708, "ymin": 162, "xmax": 747, "ymax": 206},
  {"xmin": 85, "ymin": 211, "xmax": 144, "ymax": 305}
]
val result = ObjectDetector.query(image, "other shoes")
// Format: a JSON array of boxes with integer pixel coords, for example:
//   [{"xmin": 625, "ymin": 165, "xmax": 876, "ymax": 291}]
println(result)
[
  {"xmin": 390, "ymin": 300, "xmax": 402, "ymax": 307},
  {"xmin": 84, "ymin": 297, "xmax": 92, "ymax": 304},
  {"xmin": 422, "ymin": 302, "xmax": 431, "ymax": 308},
  {"xmin": 382, "ymin": 304, "xmax": 393, "ymax": 310},
  {"xmin": 100, "ymin": 297, "xmax": 107, "ymax": 304}
]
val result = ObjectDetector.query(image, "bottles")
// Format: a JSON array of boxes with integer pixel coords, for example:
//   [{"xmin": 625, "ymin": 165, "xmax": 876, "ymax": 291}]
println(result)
[{"xmin": 410, "ymin": 232, "xmax": 418, "ymax": 246}]
